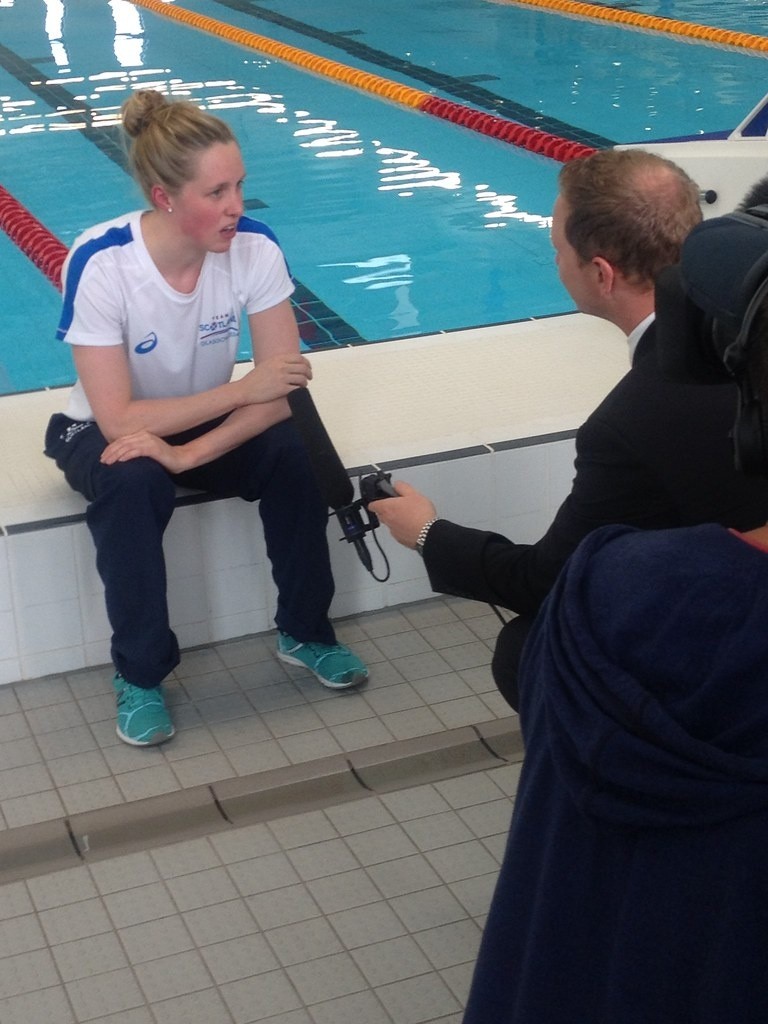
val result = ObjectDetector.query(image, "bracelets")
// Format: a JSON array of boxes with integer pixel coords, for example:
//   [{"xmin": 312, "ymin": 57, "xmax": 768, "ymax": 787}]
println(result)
[{"xmin": 416, "ymin": 518, "xmax": 440, "ymax": 554}]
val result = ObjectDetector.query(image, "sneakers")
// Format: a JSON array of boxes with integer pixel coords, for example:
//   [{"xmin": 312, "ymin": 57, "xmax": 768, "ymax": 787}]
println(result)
[
  {"xmin": 113, "ymin": 670, "xmax": 175, "ymax": 746},
  {"xmin": 276, "ymin": 626, "xmax": 370, "ymax": 690}
]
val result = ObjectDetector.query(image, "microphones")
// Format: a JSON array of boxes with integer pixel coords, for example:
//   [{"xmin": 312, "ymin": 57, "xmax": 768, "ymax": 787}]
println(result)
[{"xmin": 287, "ymin": 387, "xmax": 374, "ymax": 573}]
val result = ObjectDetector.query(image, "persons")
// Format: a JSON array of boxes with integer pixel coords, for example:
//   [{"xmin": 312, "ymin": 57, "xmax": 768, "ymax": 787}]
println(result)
[
  {"xmin": 463, "ymin": 216, "xmax": 768, "ymax": 1024},
  {"xmin": 44, "ymin": 89, "xmax": 370, "ymax": 746},
  {"xmin": 366, "ymin": 149, "xmax": 768, "ymax": 712}
]
